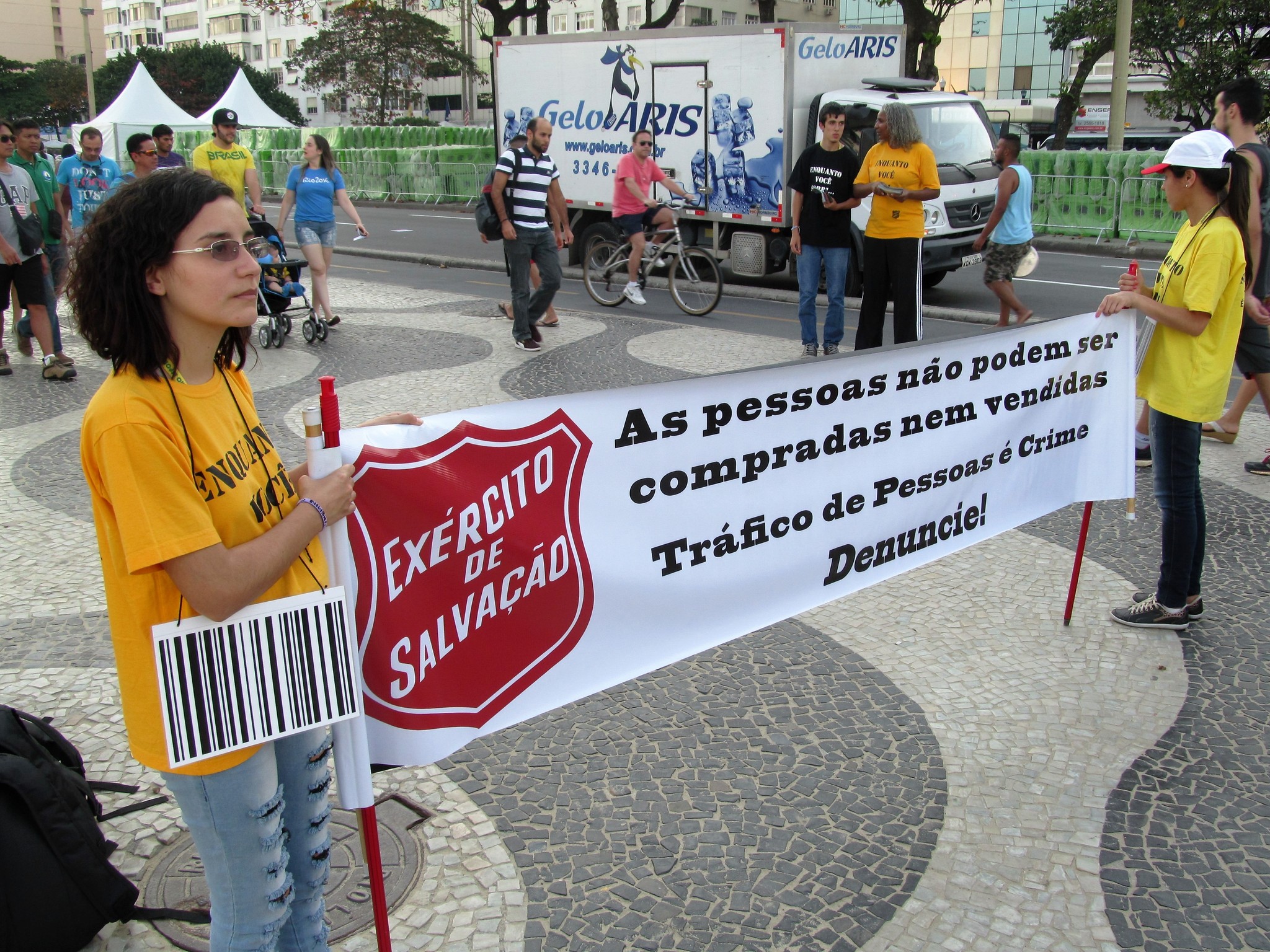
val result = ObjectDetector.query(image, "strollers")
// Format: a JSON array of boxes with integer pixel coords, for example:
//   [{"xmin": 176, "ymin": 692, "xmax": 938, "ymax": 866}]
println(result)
[{"xmin": 235, "ymin": 206, "xmax": 329, "ymax": 349}]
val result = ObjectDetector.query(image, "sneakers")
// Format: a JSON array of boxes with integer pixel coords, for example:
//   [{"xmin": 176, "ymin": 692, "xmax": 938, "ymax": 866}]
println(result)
[
  {"xmin": 642, "ymin": 246, "xmax": 666, "ymax": 267},
  {"xmin": 1131, "ymin": 592, "xmax": 1204, "ymax": 619},
  {"xmin": 1135, "ymin": 444, "xmax": 1153, "ymax": 466},
  {"xmin": 801, "ymin": 343, "xmax": 840, "ymax": 358},
  {"xmin": 1244, "ymin": 449, "xmax": 1270, "ymax": 475},
  {"xmin": 515, "ymin": 323, "xmax": 541, "ymax": 351},
  {"xmin": 0, "ymin": 323, "xmax": 76, "ymax": 380},
  {"xmin": 623, "ymin": 285, "xmax": 647, "ymax": 304},
  {"xmin": 1109, "ymin": 594, "xmax": 1189, "ymax": 629}
]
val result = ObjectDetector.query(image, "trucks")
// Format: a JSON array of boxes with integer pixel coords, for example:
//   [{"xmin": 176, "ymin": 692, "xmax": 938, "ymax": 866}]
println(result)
[{"xmin": 490, "ymin": 23, "xmax": 1012, "ymax": 299}]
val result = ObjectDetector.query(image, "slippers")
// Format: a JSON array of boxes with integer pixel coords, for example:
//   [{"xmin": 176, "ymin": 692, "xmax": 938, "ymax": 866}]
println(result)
[
  {"xmin": 536, "ymin": 320, "xmax": 560, "ymax": 326},
  {"xmin": 498, "ymin": 302, "xmax": 514, "ymax": 320}
]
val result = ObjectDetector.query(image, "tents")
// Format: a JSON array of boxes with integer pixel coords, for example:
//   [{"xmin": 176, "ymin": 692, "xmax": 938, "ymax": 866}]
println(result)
[
  {"xmin": 71, "ymin": 61, "xmax": 214, "ymax": 168},
  {"xmin": 196, "ymin": 64, "xmax": 303, "ymax": 131}
]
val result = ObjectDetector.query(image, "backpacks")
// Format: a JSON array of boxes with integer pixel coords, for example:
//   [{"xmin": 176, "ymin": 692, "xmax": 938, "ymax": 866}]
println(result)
[{"xmin": 0, "ymin": 703, "xmax": 137, "ymax": 952}]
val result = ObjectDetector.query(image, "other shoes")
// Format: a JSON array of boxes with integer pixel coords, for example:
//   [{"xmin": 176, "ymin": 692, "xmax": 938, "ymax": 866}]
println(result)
[
  {"xmin": 309, "ymin": 315, "xmax": 340, "ymax": 327},
  {"xmin": 282, "ymin": 282, "xmax": 303, "ymax": 299}
]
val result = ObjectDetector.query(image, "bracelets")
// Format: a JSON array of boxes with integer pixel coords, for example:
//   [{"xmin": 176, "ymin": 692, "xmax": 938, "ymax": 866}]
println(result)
[
  {"xmin": 499, "ymin": 219, "xmax": 509, "ymax": 224},
  {"xmin": 294, "ymin": 498, "xmax": 327, "ymax": 532},
  {"xmin": 791, "ymin": 226, "xmax": 798, "ymax": 231}
]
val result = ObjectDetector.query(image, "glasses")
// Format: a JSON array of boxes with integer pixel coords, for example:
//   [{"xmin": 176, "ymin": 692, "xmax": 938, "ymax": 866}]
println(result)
[
  {"xmin": 0, "ymin": 136, "xmax": 17, "ymax": 143},
  {"xmin": 134, "ymin": 149, "xmax": 158, "ymax": 156},
  {"xmin": 634, "ymin": 141, "xmax": 653, "ymax": 147},
  {"xmin": 81, "ymin": 142, "xmax": 102, "ymax": 152},
  {"xmin": 170, "ymin": 235, "xmax": 275, "ymax": 262}
]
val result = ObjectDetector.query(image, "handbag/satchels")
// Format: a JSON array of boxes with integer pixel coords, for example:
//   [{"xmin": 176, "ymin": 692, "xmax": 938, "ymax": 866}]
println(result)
[
  {"xmin": 17, "ymin": 212, "xmax": 44, "ymax": 256},
  {"xmin": 475, "ymin": 148, "xmax": 522, "ymax": 241},
  {"xmin": 48, "ymin": 209, "xmax": 62, "ymax": 239}
]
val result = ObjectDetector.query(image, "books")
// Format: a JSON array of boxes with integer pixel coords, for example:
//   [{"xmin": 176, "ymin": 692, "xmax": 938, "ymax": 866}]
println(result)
[{"xmin": 878, "ymin": 182, "xmax": 903, "ymax": 198}]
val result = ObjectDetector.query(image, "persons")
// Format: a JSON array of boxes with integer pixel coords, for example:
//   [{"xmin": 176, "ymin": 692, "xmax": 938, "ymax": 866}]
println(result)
[
  {"xmin": 613, "ymin": 128, "xmax": 695, "ymax": 305},
  {"xmin": 62, "ymin": 170, "xmax": 425, "ymax": 952},
  {"xmin": 480, "ymin": 134, "xmax": 563, "ymax": 326},
  {"xmin": 278, "ymin": 135, "xmax": 369, "ymax": 328},
  {"xmin": 969, "ymin": 131, "xmax": 1035, "ymax": 329},
  {"xmin": 1, "ymin": 108, "xmax": 303, "ymax": 379},
  {"xmin": 1135, "ymin": 76, "xmax": 1270, "ymax": 475},
  {"xmin": 1098, "ymin": 130, "xmax": 1254, "ymax": 629},
  {"xmin": 786, "ymin": 101, "xmax": 862, "ymax": 359},
  {"xmin": 852, "ymin": 102, "xmax": 942, "ymax": 351},
  {"xmin": 490, "ymin": 116, "xmax": 574, "ymax": 350}
]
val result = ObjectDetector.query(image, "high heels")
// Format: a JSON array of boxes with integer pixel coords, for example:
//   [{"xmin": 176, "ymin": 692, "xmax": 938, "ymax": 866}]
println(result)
[{"xmin": 1201, "ymin": 422, "xmax": 1238, "ymax": 444}]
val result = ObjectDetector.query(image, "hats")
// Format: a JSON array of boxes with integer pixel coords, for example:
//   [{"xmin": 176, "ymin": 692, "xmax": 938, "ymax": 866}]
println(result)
[
  {"xmin": 213, "ymin": 108, "xmax": 242, "ymax": 126},
  {"xmin": 1139, "ymin": 130, "xmax": 1235, "ymax": 174}
]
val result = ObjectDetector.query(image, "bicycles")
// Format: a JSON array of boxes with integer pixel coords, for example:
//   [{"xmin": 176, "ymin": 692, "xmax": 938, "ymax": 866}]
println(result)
[{"xmin": 583, "ymin": 198, "xmax": 724, "ymax": 317}]
